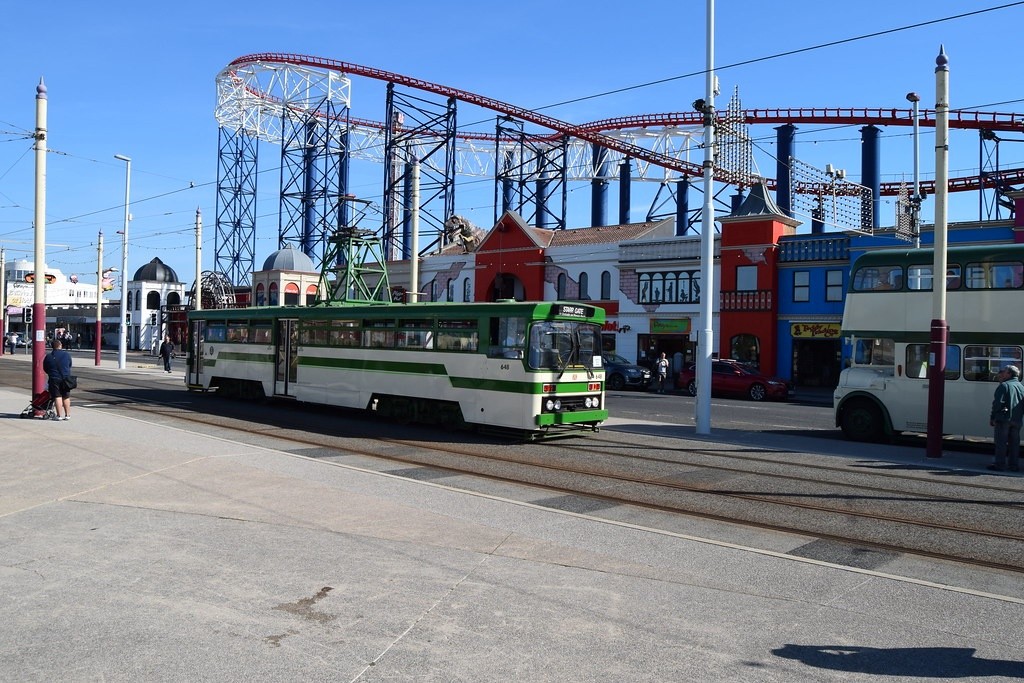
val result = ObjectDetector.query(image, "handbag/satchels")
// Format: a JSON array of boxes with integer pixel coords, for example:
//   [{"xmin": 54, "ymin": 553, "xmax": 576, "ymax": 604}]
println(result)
[
  {"xmin": 63, "ymin": 376, "xmax": 78, "ymax": 390},
  {"xmin": 172, "ymin": 353, "xmax": 176, "ymax": 359}
]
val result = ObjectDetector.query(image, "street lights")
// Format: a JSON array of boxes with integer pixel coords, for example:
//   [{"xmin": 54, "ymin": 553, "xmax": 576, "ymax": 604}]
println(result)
[{"xmin": 114, "ymin": 153, "xmax": 132, "ymax": 369}]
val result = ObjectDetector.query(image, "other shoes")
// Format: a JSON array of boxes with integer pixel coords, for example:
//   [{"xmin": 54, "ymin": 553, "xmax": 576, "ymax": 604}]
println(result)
[
  {"xmin": 1007, "ymin": 465, "xmax": 1019, "ymax": 472},
  {"xmin": 164, "ymin": 370, "xmax": 168, "ymax": 373},
  {"xmin": 987, "ymin": 463, "xmax": 1005, "ymax": 471},
  {"xmin": 168, "ymin": 369, "xmax": 172, "ymax": 373},
  {"xmin": 658, "ymin": 390, "xmax": 660, "ymax": 393},
  {"xmin": 65, "ymin": 415, "xmax": 71, "ymax": 419},
  {"xmin": 55, "ymin": 416, "xmax": 65, "ymax": 420},
  {"xmin": 662, "ymin": 390, "xmax": 664, "ymax": 393}
]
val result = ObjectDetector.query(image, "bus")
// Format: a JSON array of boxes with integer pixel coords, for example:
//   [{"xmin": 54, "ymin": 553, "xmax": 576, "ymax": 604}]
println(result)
[
  {"xmin": 188, "ymin": 304, "xmax": 608, "ymax": 441},
  {"xmin": 836, "ymin": 247, "xmax": 1020, "ymax": 442}
]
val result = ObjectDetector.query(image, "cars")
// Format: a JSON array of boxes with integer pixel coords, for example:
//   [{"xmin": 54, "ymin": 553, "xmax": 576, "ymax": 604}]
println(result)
[
  {"xmin": 679, "ymin": 357, "xmax": 786, "ymax": 400},
  {"xmin": 603, "ymin": 350, "xmax": 651, "ymax": 387}
]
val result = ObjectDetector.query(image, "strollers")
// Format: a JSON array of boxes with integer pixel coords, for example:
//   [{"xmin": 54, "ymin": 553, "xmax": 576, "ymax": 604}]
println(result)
[{"xmin": 22, "ymin": 390, "xmax": 55, "ymax": 420}]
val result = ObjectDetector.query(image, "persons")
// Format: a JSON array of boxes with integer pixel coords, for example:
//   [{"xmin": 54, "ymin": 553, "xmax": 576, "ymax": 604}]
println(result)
[
  {"xmin": 43, "ymin": 340, "xmax": 72, "ymax": 420},
  {"xmin": 3, "ymin": 332, "xmax": 19, "ymax": 354},
  {"xmin": 159, "ymin": 335, "xmax": 175, "ymax": 373},
  {"xmin": 656, "ymin": 352, "xmax": 669, "ymax": 393},
  {"xmin": 990, "ymin": 365, "xmax": 1024, "ymax": 473},
  {"xmin": 47, "ymin": 330, "xmax": 81, "ymax": 349}
]
